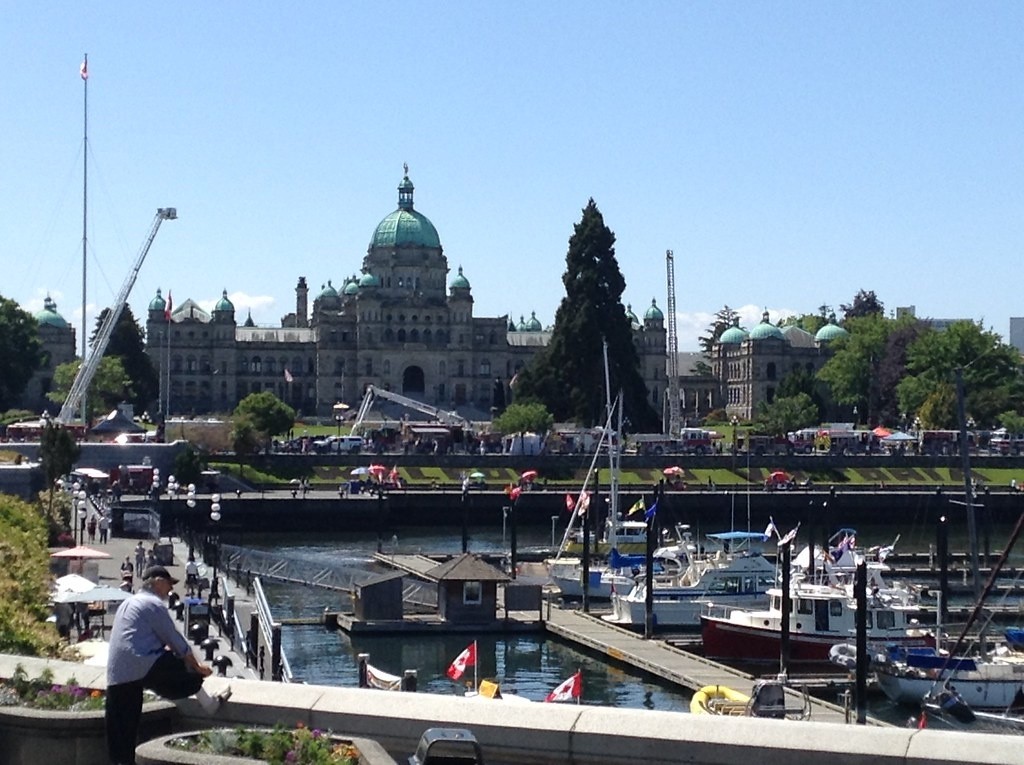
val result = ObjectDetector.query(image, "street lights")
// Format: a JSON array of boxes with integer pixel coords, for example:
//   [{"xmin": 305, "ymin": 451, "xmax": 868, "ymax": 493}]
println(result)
[
  {"xmin": 211, "ymin": 491, "xmax": 221, "ymax": 599},
  {"xmin": 71, "ymin": 482, "xmax": 87, "ymax": 549},
  {"xmin": 153, "ymin": 468, "xmax": 160, "ymax": 513},
  {"xmin": 187, "ymin": 483, "xmax": 196, "ymax": 563},
  {"xmin": 168, "ymin": 474, "xmax": 175, "ymax": 542}
]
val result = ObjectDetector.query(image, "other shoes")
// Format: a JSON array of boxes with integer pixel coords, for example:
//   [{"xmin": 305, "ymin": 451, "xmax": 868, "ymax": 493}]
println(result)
[{"xmin": 206, "ymin": 685, "xmax": 230, "ymax": 715}]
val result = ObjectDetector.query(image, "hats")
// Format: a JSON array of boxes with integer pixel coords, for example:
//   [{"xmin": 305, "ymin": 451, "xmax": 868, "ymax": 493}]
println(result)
[{"xmin": 142, "ymin": 565, "xmax": 179, "ymax": 585}]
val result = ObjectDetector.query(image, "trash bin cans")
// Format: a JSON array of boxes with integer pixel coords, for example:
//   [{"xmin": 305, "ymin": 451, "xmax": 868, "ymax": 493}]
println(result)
[
  {"xmin": 158, "ymin": 544, "xmax": 174, "ymax": 566},
  {"xmin": 183, "ymin": 600, "xmax": 211, "ymax": 642},
  {"xmin": 406, "ymin": 727, "xmax": 483, "ymax": 765}
]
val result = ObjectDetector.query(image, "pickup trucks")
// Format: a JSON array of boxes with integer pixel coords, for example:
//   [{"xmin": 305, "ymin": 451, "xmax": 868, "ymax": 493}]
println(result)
[{"xmin": 312, "ymin": 435, "xmax": 363, "ymax": 455}]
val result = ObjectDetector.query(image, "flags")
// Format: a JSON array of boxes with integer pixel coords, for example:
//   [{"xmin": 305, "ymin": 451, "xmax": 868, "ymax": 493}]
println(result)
[
  {"xmin": 565, "ymin": 492, "xmax": 591, "ymax": 516},
  {"xmin": 546, "ymin": 673, "xmax": 580, "ymax": 702},
  {"xmin": 165, "ymin": 290, "xmax": 172, "ymax": 320},
  {"xmin": 285, "ymin": 368, "xmax": 293, "ymax": 382},
  {"xmin": 765, "ymin": 523, "xmax": 796, "ymax": 547},
  {"xmin": 446, "ymin": 644, "xmax": 476, "ymax": 682},
  {"xmin": 627, "ymin": 499, "xmax": 658, "ymax": 520}
]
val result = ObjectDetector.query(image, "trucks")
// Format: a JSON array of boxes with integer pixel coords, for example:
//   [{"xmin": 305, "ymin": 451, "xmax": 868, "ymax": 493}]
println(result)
[{"xmin": 735, "ymin": 422, "xmax": 1024, "ymax": 457}]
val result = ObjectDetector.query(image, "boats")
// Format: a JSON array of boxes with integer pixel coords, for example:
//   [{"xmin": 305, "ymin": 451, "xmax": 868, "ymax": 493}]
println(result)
[
  {"xmin": 566, "ymin": 518, "xmax": 675, "ymax": 545},
  {"xmin": 789, "ymin": 546, "xmax": 890, "ymax": 591},
  {"xmin": 689, "ymin": 685, "xmax": 788, "ymax": 719},
  {"xmin": 552, "ymin": 548, "xmax": 666, "ymax": 602},
  {"xmin": 600, "ymin": 549, "xmax": 783, "ymax": 632},
  {"xmin": 872, "ymin": 650, "xmax": 1024, "ymax": 714},
  {"xmin": 700, "ymin": 581, "xmax": 939, "ymax": 670},
  {"xmin": 828, "ymin": 643, "xmax": 857, "ymax": 668},
  {"xmin": 507, "ymin": 558, "xmax": 583, "ymax": 586}
]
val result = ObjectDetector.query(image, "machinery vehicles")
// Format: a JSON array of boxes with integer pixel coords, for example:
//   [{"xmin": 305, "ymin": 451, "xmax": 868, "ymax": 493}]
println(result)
[
  {"xmin": 634, "ymin": 249, "xmax": 712, "ymax": 454},
  {"xmin": 6, "ymin": 206, "xmax": 178, "ymax": 445},
  {"xmin": 350, "ymin": 384, "xmax": 465, "ymax": 450},
  {"xmin": 554, "ymin": 425, "xmax": 618, "ymax": 454}
]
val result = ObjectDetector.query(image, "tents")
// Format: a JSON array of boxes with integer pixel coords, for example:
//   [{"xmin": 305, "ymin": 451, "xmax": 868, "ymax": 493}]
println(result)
[{"xmin": 872, "ymin": 426, "xmax": 918, "ymax": 446}]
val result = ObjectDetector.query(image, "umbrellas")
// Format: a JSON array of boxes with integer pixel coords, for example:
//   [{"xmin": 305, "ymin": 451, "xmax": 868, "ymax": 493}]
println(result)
[
  {"xmin": 350, "ymin": 466, "xmax": 372, "ymax": 479},
  {"xmin": 50, "ymin": 547, "xmax": 114, "ymax": 574},
  {"xmin": 49, "ymin": 574, "xmax": 134, "ymax": 630},
  {"xmin": 664, "ymin": 467, "xmax": 686, "ymax": 479},
  {"xmin": 471, "ymin": 472, "xmax": 485, "ymax": 478}
]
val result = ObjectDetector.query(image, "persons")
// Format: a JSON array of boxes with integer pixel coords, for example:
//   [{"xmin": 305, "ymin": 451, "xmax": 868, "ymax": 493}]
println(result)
[
  {"xmin": 121, "ymin": 542, "xmax": 157, "ymax": 577},
  {"xmin": 185, "ymin": 557, "xmax": 199, "ymax": 595},
  {"xmin": 88, "ymin": 513, "xmax": 108, "ymax": 544},
  {"xmin": 52, "ymin": 603, "xmax": 90, "ymax": 645},
  {"xmin": 790, "ymin": 474, "xmax": 796, "ymax": 482},
  {"xmin": 105, "ymin": 566, "xmax": 232, "ymax": 765},
  {"xmin": 707, "ymin": 476, "xmax": 715, "ymax": 491},
  {"xmin": 1010, "ymin": 478, "xmax": 1016, "ymax": 491},
  {"xmin": 15, "ymin": 455, "xmax": 23, "ymax": 465},
  {"xmin": 900, "ymin": 442, "xmax": 904, "ymax": 449},
  {"xmin": 111, "ymin": 481, "xmax": 122, "ymax": 500},
  {"xmin": 773, "ymin": 474, "xmax": 777, "ymax": 482},
  {"xmin": 299, "ymin": 476, "xmax": 310, "ymax": 495},
  {"xmin": 666, "ymin": 474, "xmax": 683, "ymax": 490},
  {"xmin": 358, "ymin": 476, "xmax": 371, "ymax": 491}
]
